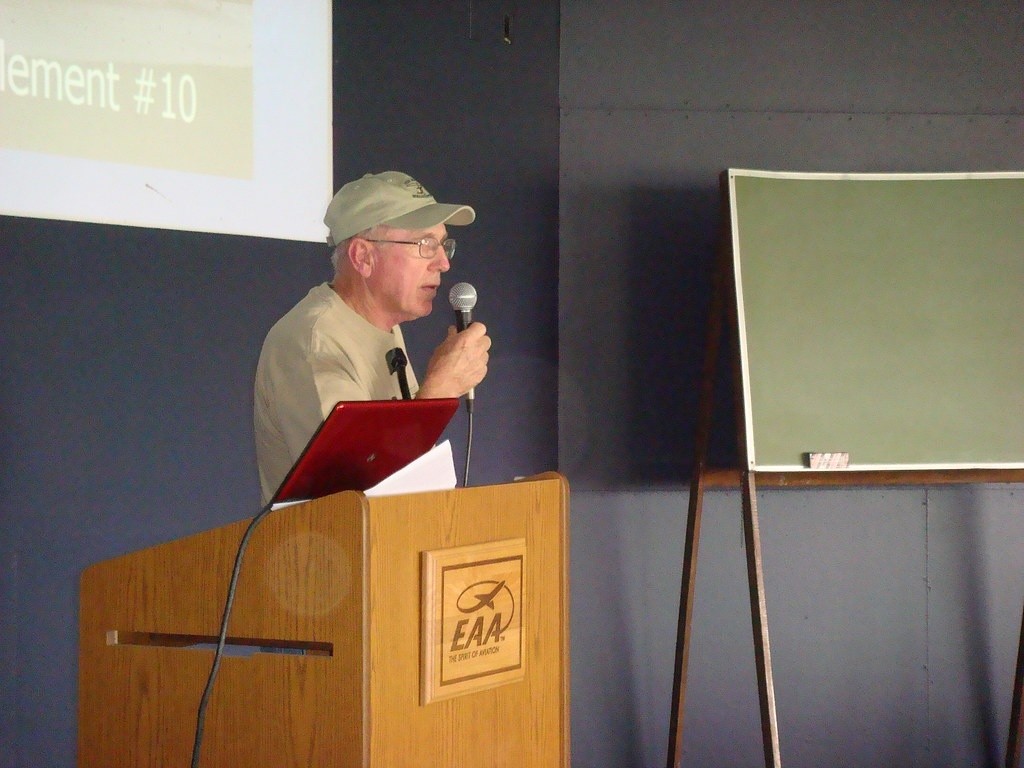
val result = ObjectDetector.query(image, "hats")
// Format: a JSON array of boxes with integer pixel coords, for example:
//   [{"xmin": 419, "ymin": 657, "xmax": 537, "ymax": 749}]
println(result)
[{"xmin": 323, "ymin": 171, "xmax": 476, "ymax": 248}]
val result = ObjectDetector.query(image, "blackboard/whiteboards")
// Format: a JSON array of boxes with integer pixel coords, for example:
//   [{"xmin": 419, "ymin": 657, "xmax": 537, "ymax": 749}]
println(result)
[{"xmin": 720, "ymin": 168, "xmax": 1024, "ymax": 473}]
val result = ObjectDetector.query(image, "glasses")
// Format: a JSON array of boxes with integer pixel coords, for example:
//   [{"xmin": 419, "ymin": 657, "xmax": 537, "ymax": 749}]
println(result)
[{"xmin": 368, "ymin": 237, "xmax": 456, "ymax": 260}]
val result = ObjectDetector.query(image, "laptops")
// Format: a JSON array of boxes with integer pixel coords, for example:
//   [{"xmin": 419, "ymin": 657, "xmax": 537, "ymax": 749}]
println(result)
[{"xmin": 267, "ymin": 396, "xmax": 460, "ymax": 511}]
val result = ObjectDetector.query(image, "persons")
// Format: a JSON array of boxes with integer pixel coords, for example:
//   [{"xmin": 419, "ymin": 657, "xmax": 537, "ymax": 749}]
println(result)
[{"xmin": 254, "ymin": 169, "xmax": 491, "ymax": 508}]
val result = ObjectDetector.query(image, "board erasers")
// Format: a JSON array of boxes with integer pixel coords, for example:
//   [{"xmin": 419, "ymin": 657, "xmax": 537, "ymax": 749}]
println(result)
[{"xmin": 809, "ymin": 451, "xmax": 849, "ymax": 470}]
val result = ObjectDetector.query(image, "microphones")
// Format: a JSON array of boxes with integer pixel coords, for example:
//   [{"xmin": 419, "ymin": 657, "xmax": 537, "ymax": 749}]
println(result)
[{"xmin": 449, "ymin": 282, "xmax": 477, "ymax": 412}]
